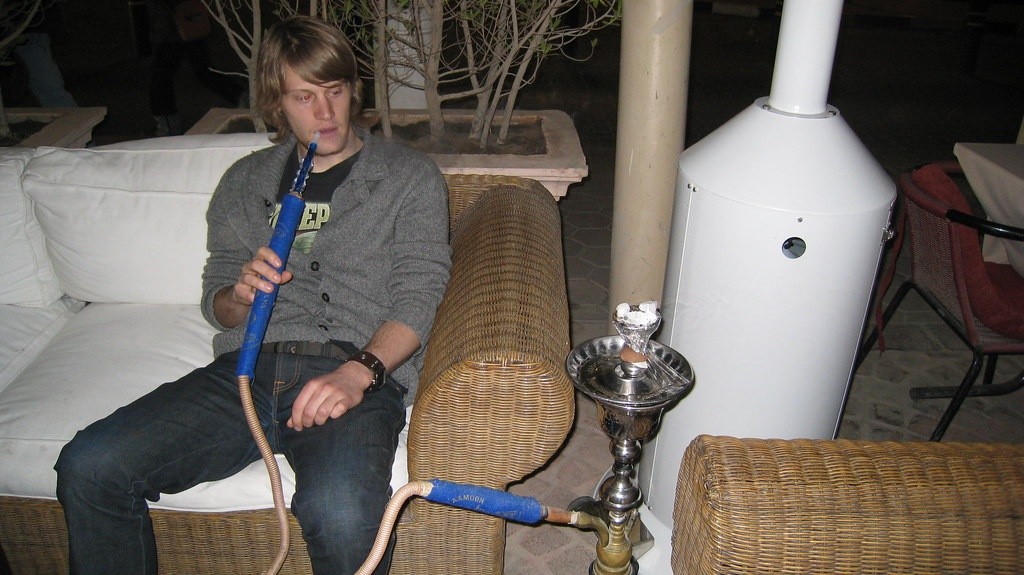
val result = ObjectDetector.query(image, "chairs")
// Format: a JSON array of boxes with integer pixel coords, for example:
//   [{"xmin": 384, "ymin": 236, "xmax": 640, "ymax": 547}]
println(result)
[
  {"xmin": 854, "ymin": 161, "xmax": 1024, "ymax": 441},
  {"xmin": 671, "ymin": 435, "xmax": 1024, "ymax": 575}
]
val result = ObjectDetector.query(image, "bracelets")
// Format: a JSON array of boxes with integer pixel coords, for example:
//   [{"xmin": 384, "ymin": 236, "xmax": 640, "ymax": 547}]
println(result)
[{"xmin": 346, "ymin": 351, "xmax": 386, "ymax": 394}]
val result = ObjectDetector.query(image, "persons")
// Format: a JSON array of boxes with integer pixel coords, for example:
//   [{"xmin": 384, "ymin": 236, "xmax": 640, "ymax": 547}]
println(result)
[
  {"xmin": 142, "ymin": 0, "xmax": 249, "ymax": 140},
  {"xmin": 52, "ymin": 17, "xmax": 452, "ymax": 574}
]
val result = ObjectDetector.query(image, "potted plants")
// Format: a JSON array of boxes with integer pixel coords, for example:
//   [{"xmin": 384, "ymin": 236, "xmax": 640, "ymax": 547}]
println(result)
[
  {"xmin": 182, "ymin": 0, "xmax": 622, "ymax": 201},
  {"xmin": 0, "ymin": 0, "xmax": 107, "ymax": 148}
]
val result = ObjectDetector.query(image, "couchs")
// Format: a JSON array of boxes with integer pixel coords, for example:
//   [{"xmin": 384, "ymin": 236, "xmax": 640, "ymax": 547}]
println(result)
[{"xmin": 0, "ymin": 134, "xmax": 575, "ymax": 575}]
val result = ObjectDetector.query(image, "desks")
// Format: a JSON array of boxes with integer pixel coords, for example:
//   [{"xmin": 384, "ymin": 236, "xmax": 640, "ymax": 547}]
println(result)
[{"xmin": 954, "ymin": 141, "xmax": 1024, "ymax": 276}]
